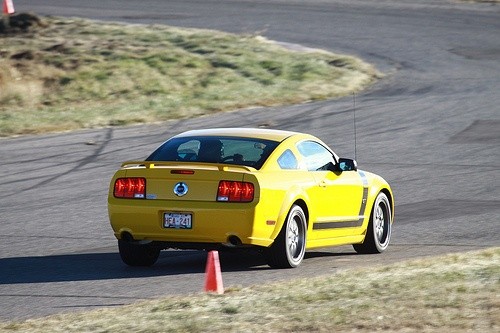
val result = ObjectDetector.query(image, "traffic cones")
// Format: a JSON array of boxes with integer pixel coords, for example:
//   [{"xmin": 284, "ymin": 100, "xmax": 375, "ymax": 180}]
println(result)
[
  {"xmin": 2, "ymin": 0, "xmax": 14, "ymax": 14},
  {"xmin": 199, "ymin": 250, "xmax": 224, "ymax": 296}
]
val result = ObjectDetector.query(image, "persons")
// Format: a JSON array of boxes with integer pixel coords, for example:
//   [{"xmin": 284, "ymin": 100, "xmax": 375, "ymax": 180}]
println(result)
[{"xmin": 197, "ymin": 138, "xmax": 247, "ymax": 166}]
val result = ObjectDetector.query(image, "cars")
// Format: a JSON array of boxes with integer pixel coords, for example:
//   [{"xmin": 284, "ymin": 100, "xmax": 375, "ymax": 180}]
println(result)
[{"xmin": 106, "ymin": 127, "xmax": 396, "ymax": 271}]
